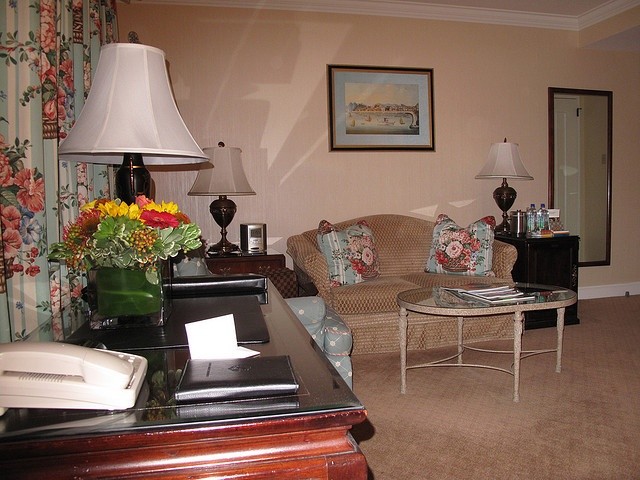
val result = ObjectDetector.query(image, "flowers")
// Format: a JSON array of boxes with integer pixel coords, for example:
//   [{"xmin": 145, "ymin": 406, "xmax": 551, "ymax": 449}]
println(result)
[{"xmin": 46, "ymin": 196, "xmax": 202, "ymax": 268}]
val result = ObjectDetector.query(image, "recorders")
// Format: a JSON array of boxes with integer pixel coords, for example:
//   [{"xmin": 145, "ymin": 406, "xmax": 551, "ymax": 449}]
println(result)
[{"xmin": 238, "ymin": 221, "xmax": 270, "ymax": 259}]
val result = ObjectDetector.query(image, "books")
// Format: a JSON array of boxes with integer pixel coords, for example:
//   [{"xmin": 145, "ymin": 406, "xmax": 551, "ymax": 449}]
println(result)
[
  {"xmin": 445, "ymin": 285, "xmax": 536, "ymax": 305},
  {"xmin": 174, "ymin": 354, "xmax": 298, "ymax": 401}
]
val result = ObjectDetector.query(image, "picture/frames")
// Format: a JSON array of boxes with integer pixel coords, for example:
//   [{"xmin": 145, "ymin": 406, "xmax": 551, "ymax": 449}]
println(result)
[{"xmin": 326, "ymin": 63, "xmax": 437, "ymax": 152}]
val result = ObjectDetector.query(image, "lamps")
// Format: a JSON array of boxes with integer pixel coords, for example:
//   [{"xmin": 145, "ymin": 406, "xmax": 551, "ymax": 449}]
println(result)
[
  {"xmin": 55, "ymin": 26, "xmax": 212, "ymax": 206},
  {"xmin": 188, "ymin": 142, "xmax": 256, "ymax": 251},
  {"xmin": 474, "ymin": 136, "xmax": 535, "ymax": 235}
]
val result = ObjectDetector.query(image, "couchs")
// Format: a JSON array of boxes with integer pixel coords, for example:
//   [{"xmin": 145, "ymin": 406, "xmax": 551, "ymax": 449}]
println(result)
[
  {"xmin": 285, "ymin": 213, "xmax": 525, "ymax": 355},
  {"xmin": 173, "ymin": 254, "xmax": 354, "ymax": 393}
]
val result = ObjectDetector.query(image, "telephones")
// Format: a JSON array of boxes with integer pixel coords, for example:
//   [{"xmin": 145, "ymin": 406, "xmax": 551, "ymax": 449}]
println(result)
[{"xmin": 0, "ymin": 341, "xmax": 147, "ymax": 416}]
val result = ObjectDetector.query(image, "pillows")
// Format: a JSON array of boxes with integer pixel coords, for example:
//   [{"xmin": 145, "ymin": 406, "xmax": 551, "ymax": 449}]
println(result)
[
  {"xmin": 316, "ymin": 219, "xmax": 382, "ymax": 290},
  {"xmin": 424, "ymin": 214, "xmax": 497, "ymax": 279},
  {"xmin": 255, "ymin": 268, "xmax": 300, "ymax": 298}
]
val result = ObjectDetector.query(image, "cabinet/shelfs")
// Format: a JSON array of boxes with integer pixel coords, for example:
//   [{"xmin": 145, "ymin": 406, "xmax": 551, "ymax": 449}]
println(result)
[
  {"xmin": 202, "ymin": 243, "xmax": 285, "ymax": 274},
  {"xmin": 1, "ymin": 274, "xmax": 369, "ymax": 480},
  {"xmin": 493, "ymin": 233, "xmax": 582, "ymax": 330}
]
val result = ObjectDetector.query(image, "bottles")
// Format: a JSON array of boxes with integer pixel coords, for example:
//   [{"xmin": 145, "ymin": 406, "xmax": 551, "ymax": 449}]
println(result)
[
  {"xmin": 526, "ymin": 202, "xmax": 537, "ymax": 237},
  {"xmin": 536, "ymin": 201, "xmax": 550, "ymax": 231}
]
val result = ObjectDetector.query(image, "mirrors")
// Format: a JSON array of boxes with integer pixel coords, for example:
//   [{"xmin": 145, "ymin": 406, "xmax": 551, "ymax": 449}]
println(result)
[{"xmin": 548, "ymin": 86, "xmax": 613, "ymax": 269}]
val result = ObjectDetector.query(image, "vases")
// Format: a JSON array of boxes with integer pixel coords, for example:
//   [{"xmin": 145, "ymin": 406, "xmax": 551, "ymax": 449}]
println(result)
[{"xmin": 87, "ymin": 263, "xmax": 172, "ymax": 331}]
